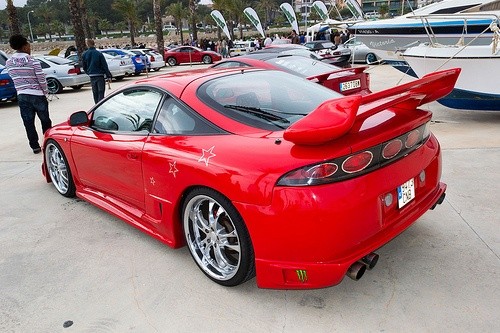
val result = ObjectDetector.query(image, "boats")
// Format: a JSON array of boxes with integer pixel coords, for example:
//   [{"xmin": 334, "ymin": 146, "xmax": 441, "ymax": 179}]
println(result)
[
  {"xmin": 399, "ymin": 14, "xmax": 500, "ymax": 111},
  {"xmin": 346, "ymin": 0, "xmax": 500, "ymax": 78}
]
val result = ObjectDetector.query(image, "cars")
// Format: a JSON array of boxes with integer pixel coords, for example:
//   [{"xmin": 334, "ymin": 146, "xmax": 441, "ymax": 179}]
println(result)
[
  {"xmin": 230, "ymin": 40, "xmax": 255, "ymax": 56},
  {"xmin": 0, "ymin": 48, "xmax": 165, "ymax": 103},
  {"xmin": 249, "ymin": 37, "xmax": 350, "ymax": 67},
  {"xmin": 163, "ymin": 46, "xmax": 222, "ymax": 66},
  {"xmin": 344, "ymin": 37, "xmax": 378, "ymax": 65},
  {"xmin": 208, "ymin": 53, "xmax": 372, "ymax": 97}
]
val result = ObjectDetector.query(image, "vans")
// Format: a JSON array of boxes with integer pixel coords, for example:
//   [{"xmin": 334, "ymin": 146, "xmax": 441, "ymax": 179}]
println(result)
[{"xmin": 162, "ymin": 25, "xmax": 177, "ymax": 32}]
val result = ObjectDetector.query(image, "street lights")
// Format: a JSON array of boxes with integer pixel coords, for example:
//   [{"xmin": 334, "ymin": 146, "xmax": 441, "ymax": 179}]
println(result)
[{"xmin": 27, "ymin": 10, "xmax": 34, "ymax": 43}]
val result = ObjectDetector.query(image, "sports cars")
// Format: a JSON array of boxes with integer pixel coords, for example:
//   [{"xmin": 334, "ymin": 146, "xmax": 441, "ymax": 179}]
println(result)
[{"xmin": 42, "ymin": 67, "xmax": 462, "ymax": 290}]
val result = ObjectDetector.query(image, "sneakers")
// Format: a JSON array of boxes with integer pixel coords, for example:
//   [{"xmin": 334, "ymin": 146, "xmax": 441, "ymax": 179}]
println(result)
[{"xmin": 33, "ymin": 147, "xmax": 41, "ymax": 154}]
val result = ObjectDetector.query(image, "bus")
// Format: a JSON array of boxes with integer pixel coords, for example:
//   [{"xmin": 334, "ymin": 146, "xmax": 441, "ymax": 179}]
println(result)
[{"xmin": 364, "ymin": 12, "xmax": 379, "ymax": 18}]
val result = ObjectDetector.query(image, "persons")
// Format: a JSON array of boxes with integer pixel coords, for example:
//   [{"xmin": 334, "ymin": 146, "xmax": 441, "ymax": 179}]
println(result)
[
  {"xmin": 82, "ymin": 39, "xmax": 112, "ymax": 105},
  {"xmin": 391, "ymin": 12, "xmax": 394, "ymax": 18},
  {"xmin": 50, "ymin": 45, "xmax": 77, "ymax": 59},
  {"xmin": 5, "ymin": 34, "xmax": 52, "ymax": 154},
  {"xmin": 99, "ymin": 41, "xmax": 146, "ymax": 50},
  {"xmin": 362, "ymin": 11, "xmax": 365, "ymax": 18},
  {"xmin": 235, "ymin": 21, "xmax": 350, "ymax": 51},
  {"xmin": 164, "ymin": 37, "xmax": 233, "ymax": 59},
  {"xmin": 327, "ymin": 12, "xmax": 336, "ymax": 20}
]
